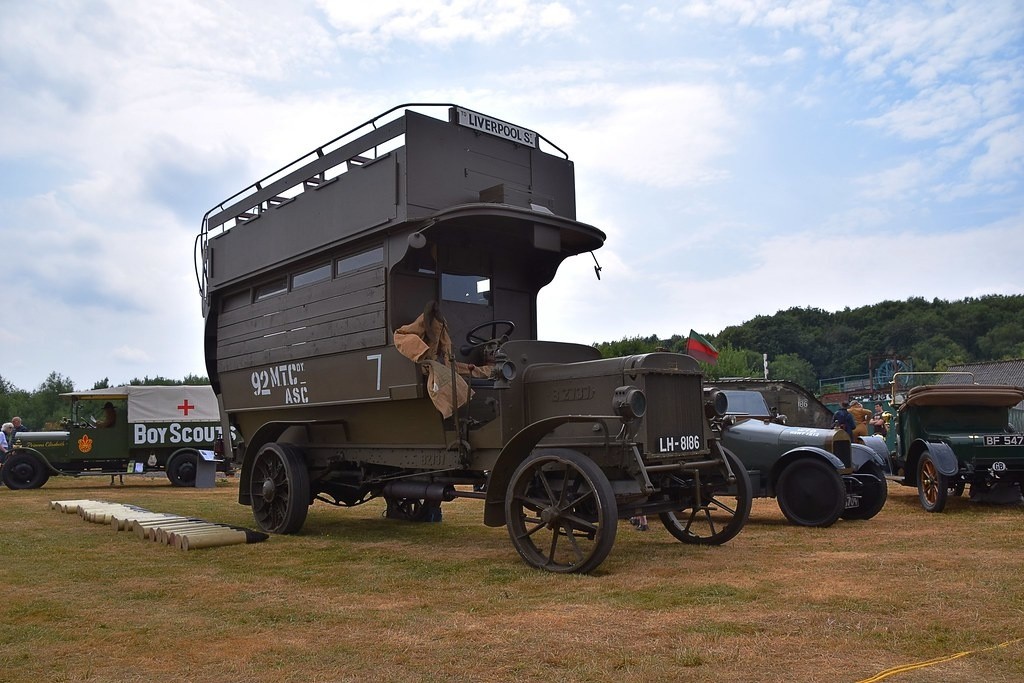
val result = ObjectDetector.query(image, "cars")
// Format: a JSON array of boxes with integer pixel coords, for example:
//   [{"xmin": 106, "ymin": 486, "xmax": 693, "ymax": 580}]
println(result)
[
  {"xmin": 857, "ymin": 372, "xmax": 1024, "ymax": 512},
  {"xmin": 712, "ymin": 390, "xmax": 887, "ymax": 528}
]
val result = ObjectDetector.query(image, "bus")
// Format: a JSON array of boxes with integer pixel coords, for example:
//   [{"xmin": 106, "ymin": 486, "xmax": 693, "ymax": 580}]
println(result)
[{"xmin": 194, "ymin": 103, "xmax": 752, "ymax": 572}]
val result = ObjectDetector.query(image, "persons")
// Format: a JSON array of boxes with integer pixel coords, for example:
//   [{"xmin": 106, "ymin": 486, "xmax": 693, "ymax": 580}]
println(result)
[
  {"xmin": 0, "ymin": 417, "xmax": 29, "ymax": 486},
  {"xmin": 832, "ymin": 400, "xmax": 891, "ymax": 444},
  {"xmin": 93, "ymin": 402, "xmax": 116, "ymax": 428}
]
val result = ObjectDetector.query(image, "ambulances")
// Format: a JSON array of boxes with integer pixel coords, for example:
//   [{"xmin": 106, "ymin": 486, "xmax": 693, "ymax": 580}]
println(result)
[{"xmin": 1, "ymin": 384, "xmax": 238, "ymax": 489}]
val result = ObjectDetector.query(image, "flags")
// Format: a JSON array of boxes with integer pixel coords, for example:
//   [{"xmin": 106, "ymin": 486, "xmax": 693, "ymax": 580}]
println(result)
[{"xmin": 687, "ymin": 330, "xmax": 719, "ymax": 367}]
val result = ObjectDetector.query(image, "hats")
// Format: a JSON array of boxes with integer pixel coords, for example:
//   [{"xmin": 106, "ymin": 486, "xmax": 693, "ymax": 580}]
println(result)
[
  {"xmin": 849, "ymin": 400, "xmax": 857, "ymax": 405},
  {"xmin": 101, "ymin": 402, "xmax": 117, "ymax": 409}
]
